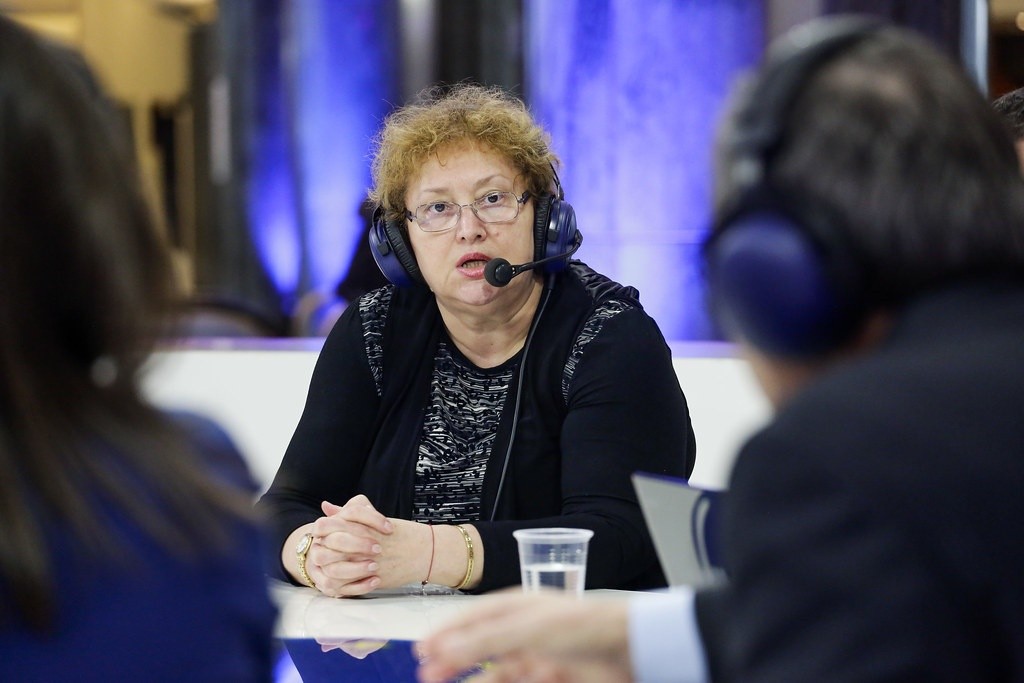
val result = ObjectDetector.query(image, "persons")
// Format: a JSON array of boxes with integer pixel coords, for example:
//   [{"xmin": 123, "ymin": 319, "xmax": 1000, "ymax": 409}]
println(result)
[
  {"xmin": 254, "ymin": 90, "xmax": 695, "ymax": 599},
  {"xmin": 0, "ymin": 18, "xmax": 283, "ymax": 682},
  {"xmin": 408, "ymin": 17, "xmax": 1023, "ymax": 683}
]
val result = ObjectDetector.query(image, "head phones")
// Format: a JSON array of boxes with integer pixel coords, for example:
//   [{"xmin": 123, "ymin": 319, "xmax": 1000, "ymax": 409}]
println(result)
[
  {"xmin": 696, "ymin": 11, "xmax": 887, "ymax": 365},
  {"xmin": 367, "ymin": 162, "xmax": 584, "ymax": 289}
]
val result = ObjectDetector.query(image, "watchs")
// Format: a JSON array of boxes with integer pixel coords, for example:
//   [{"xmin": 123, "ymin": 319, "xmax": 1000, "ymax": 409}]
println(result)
[{"xmin": 296, "ymin": 532, "xmax": 318, "ymax": 592}]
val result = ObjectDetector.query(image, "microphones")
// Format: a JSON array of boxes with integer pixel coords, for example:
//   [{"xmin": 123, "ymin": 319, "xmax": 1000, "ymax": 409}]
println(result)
[{"xmin": 485, "ymin": 242, "xmax": 582, "ymax": 287}]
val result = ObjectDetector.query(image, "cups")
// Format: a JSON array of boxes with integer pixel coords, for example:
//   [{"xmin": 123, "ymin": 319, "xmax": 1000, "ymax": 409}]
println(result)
[{"xmin": 512, "ymin": 527, "xmax": 595, "ymax": 593}]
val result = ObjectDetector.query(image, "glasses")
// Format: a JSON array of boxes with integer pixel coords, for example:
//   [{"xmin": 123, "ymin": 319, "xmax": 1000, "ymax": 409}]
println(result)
[{"xmin": 405, "ymin": 189, "xmax": 531, "ymax": 232}]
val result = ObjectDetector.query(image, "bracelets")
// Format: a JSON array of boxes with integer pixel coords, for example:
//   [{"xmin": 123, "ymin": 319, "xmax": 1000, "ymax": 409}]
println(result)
[
  {"xmin": 421, "ymin": 525, "xmax": 434, "ymax": 595},
  {"xmin": 452, "ymin": 524, "xmax": 474, "ymax": 589}
]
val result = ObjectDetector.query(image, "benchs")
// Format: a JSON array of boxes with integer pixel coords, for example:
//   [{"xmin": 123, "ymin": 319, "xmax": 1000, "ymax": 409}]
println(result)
[{"xmin": 140, "ymin": 335, "xmax": 772, "ymax": 506}]
